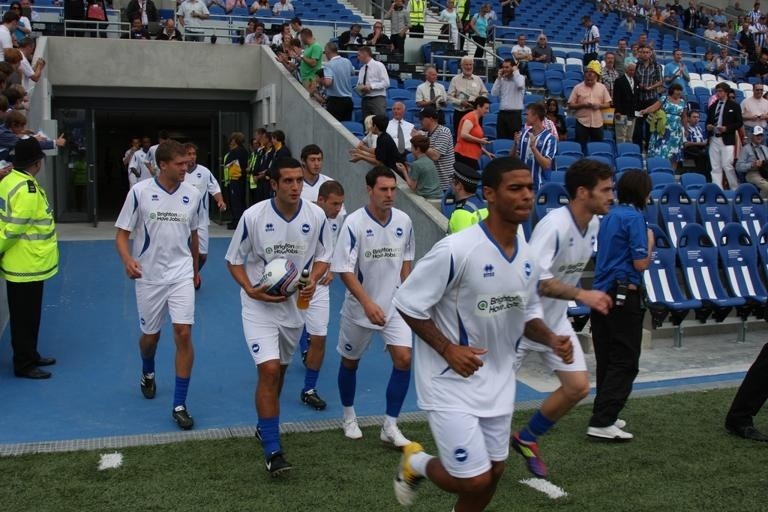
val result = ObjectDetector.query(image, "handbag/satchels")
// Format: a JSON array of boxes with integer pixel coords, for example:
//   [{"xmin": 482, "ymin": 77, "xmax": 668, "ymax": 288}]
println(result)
[
  {"xmin": 455, "ymin": 10, "xmax": 462, "ymax": 29},
  {"xmin": 463, "ymin": 14, "xmax": 479, "ymax": 34},
  {"xmin": 646, "ymin": 96, "xmax": 668, "ymax": 127}
]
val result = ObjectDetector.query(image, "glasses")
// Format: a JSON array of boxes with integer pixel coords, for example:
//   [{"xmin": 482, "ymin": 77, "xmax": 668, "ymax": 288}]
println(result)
[
  {"xmin": 728, "ymin": 96, "xmax": 736, "ymax": 100},
  {"xmin": 756, "ymin": 89, "xmax": 763, "ymax": 92}
]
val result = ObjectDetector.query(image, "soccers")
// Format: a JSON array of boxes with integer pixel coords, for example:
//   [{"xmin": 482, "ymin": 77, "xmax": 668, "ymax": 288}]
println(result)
[{"xmin": 260, "ymin": 257, "xmax": 300, "ymax": 298}]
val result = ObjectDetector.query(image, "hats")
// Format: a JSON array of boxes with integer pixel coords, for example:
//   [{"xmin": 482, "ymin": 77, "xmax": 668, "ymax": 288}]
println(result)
[
  {"xmin": 12, "ymin": 134, "xmax": 45, "ymax": 167},
  {"xmin": 447, "ymin": 160, "xmax": 482, "ymax": 186},
  {"xmin": 752, "ymin": 126, "xmax": 764, "ymax": 136}
]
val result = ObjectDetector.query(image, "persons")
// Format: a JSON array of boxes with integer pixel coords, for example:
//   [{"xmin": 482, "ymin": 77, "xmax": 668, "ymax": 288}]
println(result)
[
  {"xmin": 85, "ymin": 0, "xmax": 113, "ymax": 37},
  {"xmin": 624, "ymin": 44, "xmax": 640, "ymax": 69},
  {"xmin": 740, "ymin": 84, "xmax": 768, "ymax": 146},
  {"xmin": 725, "ymin": 345, "xmax": 768, "ymax": 444},
  {"xmin": 394, "ymin": 156, "xmax": 573, "ymax": 512},
  {"xmin": 386, "ymin": 102, "xmax": 415, "ymax": 154},
  {"xmin": 8, "ymin": 2, "xmax": 31, "ymax": 46},
  {"xmin": 144, "ymin": 132, "xmax": 172, "ymax": 177},
  {"xmin": 484, "ymin": 4, "xmax": 500, "ymax": 44},
  {"xmin": 453, "ymin": 97, "xmax": 495, "ymax": 171},
  {"xmin": 0, "ymin": 165, "xmax": 12, "ymax": 180},
  {"xmin": 184, "ymin": 144, "xmax": 227, "ymax": 291},
  {"xmin": 640, "ymin": 84, "xmax": 686, "ymax": 172},
  {"xmin": 0, "ymin": 111, "xmax": 66, "ymax": 149},
  {"xmin": 664, "ymin": 49, "xmax": 690, "ymax": 96},
  {"xmin": 599, "ymin": 52, "xmax": 619, "ymax": 139},
  {"xmin": 470, "ymin": 7, "xmax": 489, "ymax": 64},
  {"xmin": 532, "ymin": 34, "xmax": 557, "ymax": 63},
  {"xmin": 384, "ymin": 0, "xmax": 412, "ymax": 64},
  {"xmin": 509, "ymin": 103, "xmax": 558, "ymax": 194},
  {"xmin": 447, "ymin": 55, "xmax": 490, "ymax": 143},
  {"xmin": 681, "ymin": 111, "xmax": 712, "ymax": 183},
  {"xmin": 567, "ymin": 69, "xmax": 613, "ymax": 151},
  {"xmin": 272, "ymin": 23, "xmax": 290, "ymax": 46},
  {"xmin": 51, "ymin": 0, "xmax": 85, "ymax": 37},
  {"xmin": 249, "ymin": 0, "xmax": 270, "ymax": 17},
  {"xmin": 120, "ymin": 15, "xmax": 151, "ymax": 39},
  {"xmin": 224, "ymin": 128, "xmax": 291, "ymax": 230},
  {"xmin": 638, "ymin": 33, "xmax": 657, "ymax": 67},
  {"xmin": 240, "ymin": 18, "xmax": 259, "ymax": 35},
  {"xmin": 308, "ymin": 68, "xmax": 327, "ymax": 110},
  {"xmin": 684, "ymin": 1, "xmax": 699, "ymax": 35},
  {"xmin": 635, "ymin": 46, "xmax": 663, "ymax": 161},
  {"xmin": 613, "ymin": 62, "xmax": 643, "ymax": 143},
  {"xmin": 126, "ymin": 0, "xmax": 159, "ymax": 29},
  {"xmin": 21, "ymin": 128, "xmax": 36, "ymax": 134},
  {"xmin": 20, "ymin": 0, "xmax": 42, "ymax": 37},
  {"xmin": 614, "ymin": 39, "xmax": 630, "ymax": 76},
  {"xmin": 366, "ymin": 21, "xmax": 395, "ymax": 54},
  {"xmin": 546, "ymin": 98, "xmax": 567, "ymax": 141},
  {"xmin": 736, "ymin": 125, "xmax": 768, "ymax": 200},
  {"xmin": 510, "ymin": 160, "xmax": 616, "ymax": 479},
  {"xmin": 275, "ymin": 38, "xmax": 304, "ymax": 84},
  {"xmin": 439, "ymin": 0, "xmax": 458, "ymax": 51},
  {"xmin": 206, "ymin": 0, "xmax": 226, "ymax": 9},
  {"xmin": 338, "ymin": 24, "xmax": 363, "ymax": 57},
  {"xmin": 224, "ymin": 157, "xmax": 331, "ymax": 478},
  {"xmin": 728, "ymin": 88, "xmax": 736, "ymax": 102},
  {"xmin": 226, "ymin": 0, "xmax": 247, "ymax": 13},
  {"xmin": 586, "ymin": 169, "xmax": 655, "ymax": 441},
  {"xmin": 128, "ymin": 137, "xmax": 153, "ymax": 190},
  {"xmin": 411, "ymin": 107, "xmax": 456, "ymax": 194},
  {"xmin": 705, "ymin": 83, "xmax": 743, "ymax": 191},
  {"xmin": 0, "ymin": 135, "xmax": 60, "ymax": 379},
  {"xmin": 415, "ymin": 67, "xmax": 448, "ymax": 126},
  {"xmin": 510, "ymin": 34, "xmax": 532, "ymax": 75},
  {"xmin": 349, "ymin": 116, "xmax": 401, "ymax": 171},
  {"xmin": 579, "ymin": 15, "xmax": 601, "ymax": 72},
  {"xmin": 322, "ymin": 42, "xmax": 355, "ymax": 122},
  {"xmin": 288, "ymin": 29, "xmax": 323, "ymax": 85},
  {"xmin": 499, "ymin": 0, "xmax": 521, "ymax": 26},
  {"xmin": 245, "ymin": 23, "xmax": 270, "ymax": 45},
  {"xmin": 298, "ymin": 145, "xmax": 347, "ymax": 369},
  {"xmin": 524, "ymin": 100, "xmax": 558, "ymax": 171},
  {"xmin": 396, "ymin": 135, "xmax": 444, "ymax": 200},
  {"xmin": 115, "ymin": 140, "xmax": 202, "ymax": 429},
  {"xmin": 595, "ymin": 0, "xmax": 683, "ymax": 36},
  {"xmin": 447, "ymin": 163, "xmax": 488, "ymax": 236},
  {"xmin": 357, "ymin": 46, "xmax": 391, "ymax": 130},
  {"xmin": 355, "ymin": 115, "xmax": 378, "ymax": 154},
  {"xmin": 407, "ymin": 0, "xmax": 426, "ymax": 38},
  {"xmin": 277, "ymin": 34, "xmax": 293, "ymax": 54},
  {"xmin": 272, "ymin": 0, "xmax": 294, "ymax": 16},
  {"xmin": 0, "ymin": 49, "xmax": 28, "ymax": 111},
  {"xmin": 177, "ymin": 0, "xmax": 210, "ymax": 41},
  {"xmin": 451, "ymin": 0, "xmax": 471, "ymax": 51},
  {"xmin": 123, "ymin": 139, "xmax": 141, "ymax": 165},
  {"xmin": 329, "ymin": 165, "xmax": 413, "ymax": 447},
  {"xmin": 69, "ymin": 148, "xmax": 90, "ymax": 213},
  {"xmin": 491, "ymin": 58, "xmax": 526, "ymax": 140},
  {"xmin": 0, "ymin": 112, "xmax": 47, "ymax": 141},
  {"xmin": 18, "ymin": 37, "xmax": 46, "ymax": 90},
  {"xmin": 700, "ymin": 0, "xmax": 768, "ymax": 83},
  {"xmin": 156, "ymin": 18, "xmax": 182, "ymax": 41},
  {"xmin": 0, "ymin": 12, "xmax": 20, "ymax": 61},
  {"xmin": 636, "ymin": 45, "xmax": 645, "ymax": 68},
  {"xmin": 300, "ymin": 181, "xmax": 345, "ymax": 410},
  {"xmin": 291, "ymin": 17, "xmax": 317, "ymax": 48}
]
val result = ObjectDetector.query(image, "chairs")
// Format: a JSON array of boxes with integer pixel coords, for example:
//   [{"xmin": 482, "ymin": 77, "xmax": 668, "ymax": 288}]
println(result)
[
  {"xmin": 718, "ymin": 222, "xmax": 768, "ymax": 321},
  {"xmin": 522, "ymin": 213, "xmax": 532, "ymax": 243},
  {"xmin": 591, "ymin": 199, "xmax": 616, "ymax": 258},
  {"xmin": 526, "ymin": 57, "xmax": 585, "ymax": 99},
  {"xmin": 534, "ymin": 182, "xmax": 570, "ymax": 221},
  {"xmin": 757, "ymin": 222, "xmax": 768, "ymax": 286},
  {"xmin": 208, "ymin": 0, "xmax": 370, "ymax": 28},
  {"xmin": 616, "ymin": 143, "xmax": 640, "ymax": 156},
  {"xmin": 656, "ymin": 57, "xmax": 768, "ymax": 140},
  {"xmin": 471, "ymin": 0, "xmax": 743, "ymax": 58},
  {"xmin": 441, "ymin": 184, "xmax": 483, "ymax": 221},
  {"xmin": 520, "ymin": 94, "xmax": 613, "ymax": 138},
  {"xmin": 696, "ymin": 183, "xmax": 737, "ymax": 247},
  {"xmin": 649, "ymin": 172, "xmax": 675, "ymax": 199},
  {"xmin": 659, "ymin": 184, "xmax": 697, "ymax": 255},
  {"xmin": 567, "ymin": 281, "xmax": 590, "ymax": 332},
  {"xmin": 677, "ymin": 223, "xmax": 746, "ymax": 323},
  {"xmin": 158, "ymin": 8, "xmax": 175, "ymax": 19},
  {"xmin": 0, "ymin": 0, "xmax": 115, "ymax": 16},
  {"xmin": 557, "ymin": 150, "xmax": 584, "ymax": 166},
  {"xmin": 646, "ymin": 157, "xmax": 675, "ymax": 173},
  {"xmin": 407, "ymin": 151, "xmax": 415, "ymax": 162},
  {"xmin": 643, "ymin": 223, "xmax": 702, "ymax": 329},
  {"xmin": 330, "ymin": 38, "xmax": 500, "ymax": 138},
  {"xmin": 615, "ymin": 168, "xmax": 624, "ymax": 197},
  {"xmin": 551, "ymin": 166, "xmax": 569, "ymax": 182},
  {"xmin": 480, "ymin": 139, "xmax": 515, "ymax": 169},
  {"xmin": 585, "ymin": 152, "xmax": 614, "ymax": 169},
  {"xmin": 680, "ymin": 173, "xmax": 707, "ymax": 199},
  {"xmin": 732, "ymin": 183, "xmax": 768, "ymax": 241},
  {"xmin": 557, "ymin": 141, "xmax": 582, "ymax": 154},
  {"xmin": 642, "ymin": 196, "xmax": 658, "ymax": 225},
  {"xmin": 614, "ymin": 152, "xmax": 644, "ymax": 170},
  {"xmin": 585, "ymin": 139, "xmax": 615, "ymax": 155},
  {"xmin": 431, "ymin": 0, "xmax": 446, "ymax": 10}
]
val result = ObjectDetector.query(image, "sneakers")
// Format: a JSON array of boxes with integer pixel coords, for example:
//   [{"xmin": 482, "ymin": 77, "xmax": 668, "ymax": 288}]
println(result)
[
  {"xmin": 586, "ymin": 425, "xmax": 634, "ymax": 441},
  {"xmin": 140, "ymin": 369, "xmax": 157, "ymax": 399},
  {"xmin": 378, "ymin": 426, "xmax": 411, "ymax": 448},
  {"xmin": 172, "ymin": 404, "xmax": 193, "ymax": 430},
  {"xmin": 342, "ymin": 417, "xmax": 364, "ymax": 439},
  {"xmin": 614, "ymin": 417, "xmax": 627, "ymax": 428},
  {"xmin": 255, "ymin": 426, "xmax": 285, "ymax": 451},
  {"xmin": 511, "ymin": 430, "xmax": 549, "ymax": 479},
  {"xmin": 392, "ymin": 441, "xmax": 426, "ymax": 507},
  {"xmin": 725, "ymin": 421, "xmax": 768, "ymax": 442},
  {"xmin": 300, "ymin": 388, "xmax": 326, "ymax": 411},
  {"xmin": 263, "ymin": 452, "xmax": 294, "ymax": 477}
]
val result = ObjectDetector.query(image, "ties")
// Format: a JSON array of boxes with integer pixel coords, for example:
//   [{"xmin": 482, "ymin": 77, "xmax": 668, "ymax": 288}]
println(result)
[
  {"xmin": 397, "ymin": 122, "xmax": 405, "ymax": 154},
  {"xmin": 363, "ymin": 65, "xmax": 368, "ymax": 85},
  {"xmin": 715, "ymin": 102, "xmax": 724, "ymax": 126},
  {"xmin": 430, "ymin": 84, "xmax": 436, "ymax": 101}
]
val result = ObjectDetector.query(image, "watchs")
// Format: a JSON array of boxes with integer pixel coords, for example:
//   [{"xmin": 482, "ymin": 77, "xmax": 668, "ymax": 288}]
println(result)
[{"xmin": 299, "ymin": 54, "xmax": 304, "ymax": 59}]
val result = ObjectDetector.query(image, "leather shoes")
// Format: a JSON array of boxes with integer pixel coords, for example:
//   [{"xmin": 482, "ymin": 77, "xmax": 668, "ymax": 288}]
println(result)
[
  {"xmin": 35, "ymin": 356, "xmax": 56, "ymax": 367},
  {"xmin": 16, "ymin": 367, "xmax": 52, "ymax": 379}
]
